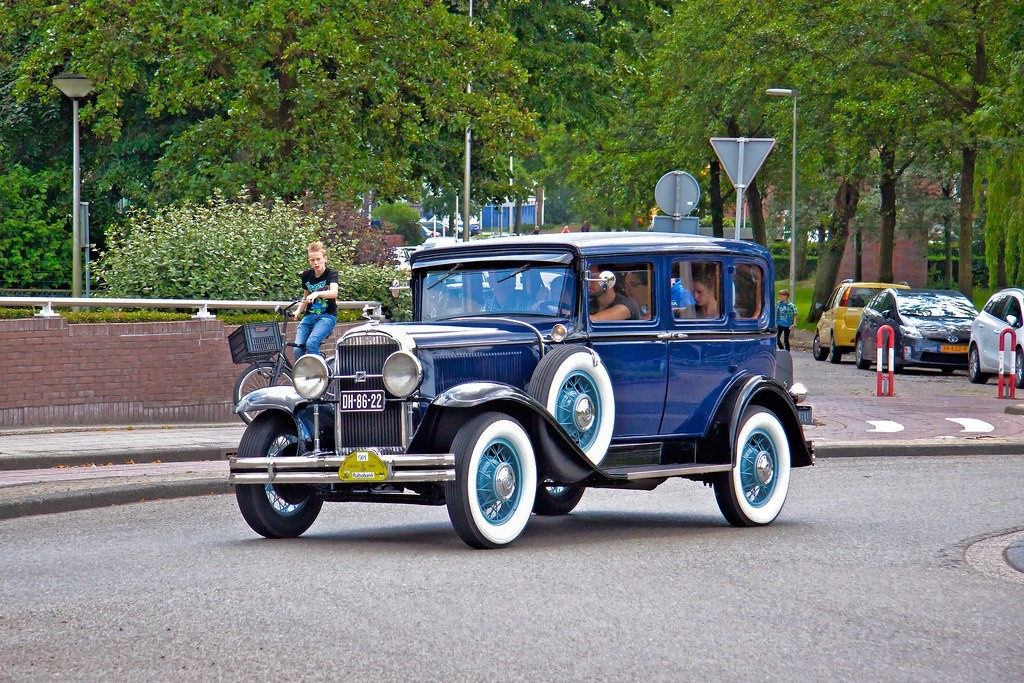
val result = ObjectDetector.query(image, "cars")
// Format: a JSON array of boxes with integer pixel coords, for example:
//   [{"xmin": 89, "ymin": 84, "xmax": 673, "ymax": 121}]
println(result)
[
  {"xmin": 966, "ymin": 287, "xmax": 1024, "ymax": 389},
  {"xmin": 419, "ymin": 218, "xmax": 479, "ymax": 240},
  {"xmin": 854, "ymin": 286, "xmax": 980, "ymax": 375},
  {"xmin": 226, "ymin": 231, "xmax": 816, "ymax": 550}
]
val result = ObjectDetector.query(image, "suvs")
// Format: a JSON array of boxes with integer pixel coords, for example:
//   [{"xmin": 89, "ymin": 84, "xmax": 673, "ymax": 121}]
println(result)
[{"xmin": 811, "ymin": 278, "xmax": 912, "ymax": 362}]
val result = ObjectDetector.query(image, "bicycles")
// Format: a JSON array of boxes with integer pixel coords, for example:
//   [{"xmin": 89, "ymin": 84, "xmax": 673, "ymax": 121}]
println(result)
[{"xmin": 228, "ymin": 296, "xmax": 336, "ymax": 425}]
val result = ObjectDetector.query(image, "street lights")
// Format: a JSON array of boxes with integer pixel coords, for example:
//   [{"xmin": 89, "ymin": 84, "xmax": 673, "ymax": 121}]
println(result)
[
  {"xmin": 766, "ymin": 88, "xmax": 795, "ymax": 339},
  {"xmin": 52, "ymin": 75, "xmax": 94, "ymax": 310}
]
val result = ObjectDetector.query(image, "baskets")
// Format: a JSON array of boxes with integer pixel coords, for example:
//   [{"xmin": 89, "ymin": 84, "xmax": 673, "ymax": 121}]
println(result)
[{"xmin": 228, "ymin": 322, "xmax": 283, "ymax": 363}]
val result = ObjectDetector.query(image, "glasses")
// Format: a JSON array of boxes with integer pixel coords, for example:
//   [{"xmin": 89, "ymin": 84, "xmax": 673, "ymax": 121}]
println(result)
[
  {"xmin": 620, "ymin": 281, "xmax": 646, "ymax": 291},
  {"xmin": 308, "ymin": 255, "xmax": 324, "ymax": 262}
]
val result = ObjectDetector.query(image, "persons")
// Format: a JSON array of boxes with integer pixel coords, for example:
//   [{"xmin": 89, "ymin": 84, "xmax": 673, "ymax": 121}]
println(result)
[
  {"xmin": 683, "ymin": 275, "xmax": 717, "ymax": 317},
  {"xmin": 562, "ymin": 226, "xmax": 570, "ymax": 233},
  {"xmin": 775, "ymin": 289, "xmax": 797, "ymax": 353},
  {"xmin": 532, "ymin": 265, "xmax": 639, "ymax": 321},
  {"xmin": 481, "ymin": 271, "xmax": 549, "ymax": 312},
  {"xmin": 531, "ymin": 226, "xmax": 539, "ymax": 235},
  {"xmin": 581, "ymin": 220, "xmax": 589, "ymax": 232},
  {"xmin": 623, "ymin": 271, "xmax": 649, "ymax": 320},
  {"xmin": 292, "ymin": 242, "xmax": 339, "ymax": 362}
]
